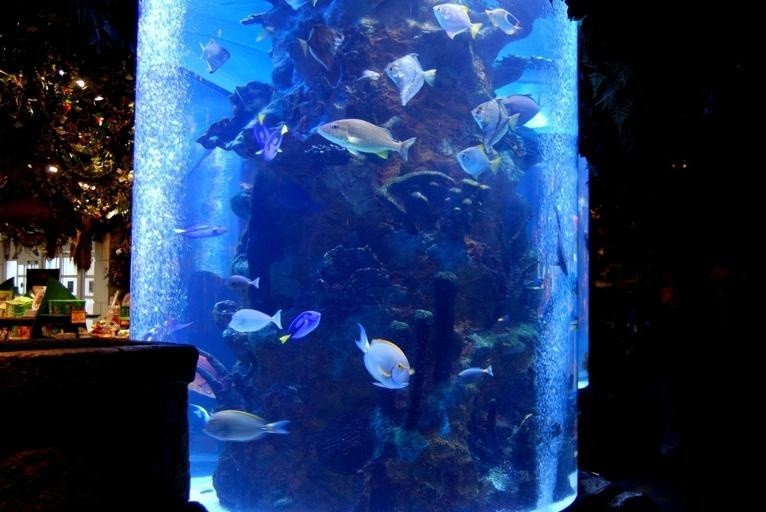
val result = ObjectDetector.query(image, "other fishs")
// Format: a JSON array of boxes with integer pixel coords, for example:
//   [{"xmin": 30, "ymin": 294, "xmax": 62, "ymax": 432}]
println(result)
[{"xmin": 174, "ymin": 3, "xmax": 576, "ymax": 492}]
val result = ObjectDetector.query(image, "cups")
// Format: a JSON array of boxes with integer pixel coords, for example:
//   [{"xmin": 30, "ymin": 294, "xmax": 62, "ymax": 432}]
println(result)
[{"xmin": 106, "ymin": 305, "xmax": 121, "ymax": 331}]
